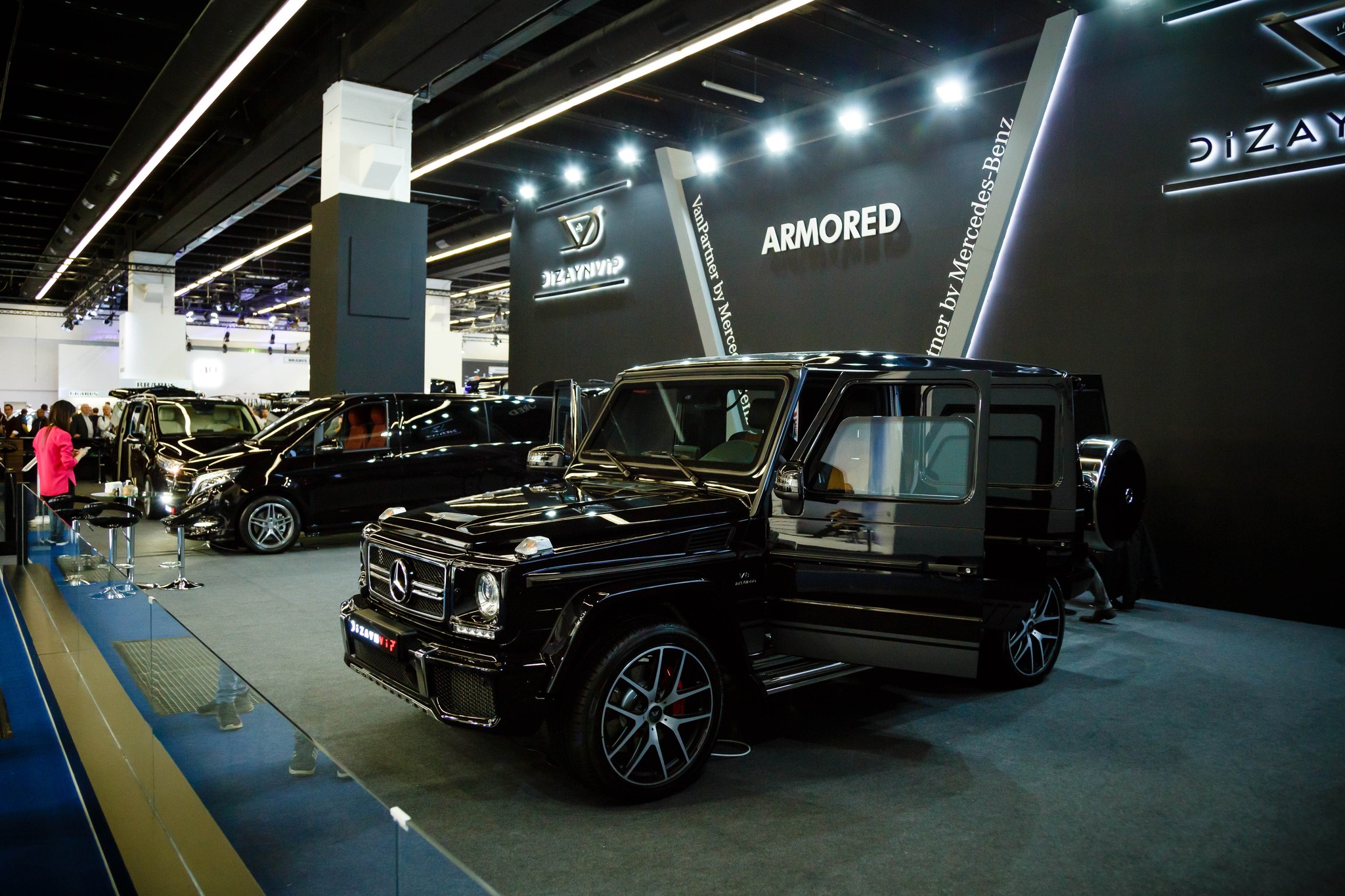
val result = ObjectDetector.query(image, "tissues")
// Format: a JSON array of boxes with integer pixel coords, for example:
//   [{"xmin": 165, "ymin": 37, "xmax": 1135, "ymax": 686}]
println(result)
[{"xmin": 123, "ymin": 479, "xmax": 134, "ymax": 497}]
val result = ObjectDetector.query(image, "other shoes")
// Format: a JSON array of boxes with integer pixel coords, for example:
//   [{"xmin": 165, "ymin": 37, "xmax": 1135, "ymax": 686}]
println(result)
[
  {"xmin": 46, "ymin": 537, "xmax": 56, "ymax": 543},
  {"xmin": 56, "ymin": 537, "xmax": 69, "ymax": 546}
]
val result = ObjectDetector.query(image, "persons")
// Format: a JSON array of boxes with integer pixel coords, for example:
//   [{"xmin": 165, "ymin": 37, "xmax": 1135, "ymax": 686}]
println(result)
[
  {"xmin": 250, "ymin": 407, "xmax": 268, "ymax": 429},
  {"xmin": 0, "ymin": 399, "xmax": 113, "ymax": 580},
  {"xmin": 325, "ymin": 416, "xmax": 342, "ymax": 439},
  {"xmin": 1062, "ymin": 555, "xmax": 1142, "ymax": 623}
]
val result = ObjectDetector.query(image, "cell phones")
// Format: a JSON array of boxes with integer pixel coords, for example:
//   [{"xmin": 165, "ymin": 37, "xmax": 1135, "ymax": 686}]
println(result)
[{"xmin": 76, "ymin": 447, "xmax": 91, "ymax": 454}]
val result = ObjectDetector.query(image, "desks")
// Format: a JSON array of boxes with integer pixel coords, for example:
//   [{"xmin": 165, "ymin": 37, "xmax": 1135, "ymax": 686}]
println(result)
[{"xmin": 92, "ymin": 491, "xmax": 172, "ymax": 592}]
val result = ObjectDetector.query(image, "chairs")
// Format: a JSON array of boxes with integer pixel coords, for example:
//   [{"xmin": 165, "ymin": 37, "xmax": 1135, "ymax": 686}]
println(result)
[
  {"xmin": 206, "ymin": 408, "xmax": 233, "ymax": 432},
  {"xmin": 728, "ymin": 399, "xmax": 785, "ymax": 441},
  {"xmin": 345, "ymin": 407, "xmax": 369, "ymax": 450},
  {"xmin": 158, "ymin": 408, "xmax": 182, "ymax": 434},
  {"xmin": 367, "ymin": 404, "xmax": 387, "ymax": 448}
]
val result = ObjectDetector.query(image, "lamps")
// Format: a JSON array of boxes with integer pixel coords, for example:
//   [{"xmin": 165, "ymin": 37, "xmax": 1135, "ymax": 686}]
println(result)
[
  {"xmin": 268, "ymin": 346, "xmax": 272, "ymax": 355},
  {"xmin": 294, "ymin": 345, "xmax": 300, "ymax": 353},
  {"xmin": 305, "ymin": 345, "xmax": 310, "ymax": 352},
  {"xmin": 284, "ymin": 344, "xmax": 287, "ymax": 354},
  {"xmin": 61, "ymin": 278, "xmax": 125, "ymax": 332},
  {"xmin": 269, "ymin": 334, "xmax": 275, "ymax": 344},
  {"xmin": 492, "ymin": 333, "xmax": 501, "ymax": 345},
  {"xmin": 495, "ymin": 305, "xmax": 502, "ymax": 316},
  {"xmin": 223, "ymin": 344, "xmax": 227, "ymax": 352},
  {"xmin": 223, "ymin": 331, "xmax": 229, "ymax": 342},
  {"xmin": 187, "ymin": 342, "xmax": 193, "ymax": 352},
  {"xmin": 237, "ymin": 311, "xmax": 247, "ymax": 326},
  {"xmin": 213, "ymin": 294, "xmax": 222, "ymax": 307}
]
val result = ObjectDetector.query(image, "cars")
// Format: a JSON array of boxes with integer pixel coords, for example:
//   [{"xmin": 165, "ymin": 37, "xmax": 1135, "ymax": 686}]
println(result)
[
  {"xmin": 464, "ymin": 373, "xmax": 509, "ymax": 395},
  {"xmin": 258, "ymin": 390, "xmax": 310, "ymax": 443},
  {"xmin": 108, "ymin": 385, "xmax": 263, "ymax": 520}
]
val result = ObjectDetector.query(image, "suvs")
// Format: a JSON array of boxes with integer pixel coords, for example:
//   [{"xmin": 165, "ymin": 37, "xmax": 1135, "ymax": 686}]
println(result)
[
  {"xmin": 160, "ymin": 391, "xmax": 588, "ymax": 555},
  {"xmin": 337, "ymin": 349, "xmax": 1147, "ymax": 805}
]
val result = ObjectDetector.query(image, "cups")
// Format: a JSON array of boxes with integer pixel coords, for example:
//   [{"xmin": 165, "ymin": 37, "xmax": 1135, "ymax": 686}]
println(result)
[{"xmin": 123, "ymin": 485, "xmax": 133, "ymax": 497}]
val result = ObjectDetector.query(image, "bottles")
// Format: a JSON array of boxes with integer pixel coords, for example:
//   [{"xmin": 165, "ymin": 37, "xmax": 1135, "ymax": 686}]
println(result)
[{"xmin": 133, "ymin": 485, "xmax": 138, "ymax": 497}]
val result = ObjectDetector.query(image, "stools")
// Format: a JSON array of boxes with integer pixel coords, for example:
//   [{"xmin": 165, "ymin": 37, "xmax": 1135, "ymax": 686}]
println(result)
[
  {"xmin": 0, "ymin": 441, "xmax": 18, "ymax": 468},
  {"xmin": 48, "ymin": 495, "xmax": 102, "ymax": 587},
  {"xmin": 82, "ymin": 501, "xmax": 143, "ymax": 600},
  {"xmin": 160, "ymin": 498, "xmax": 212, "ymax": 590}
]
